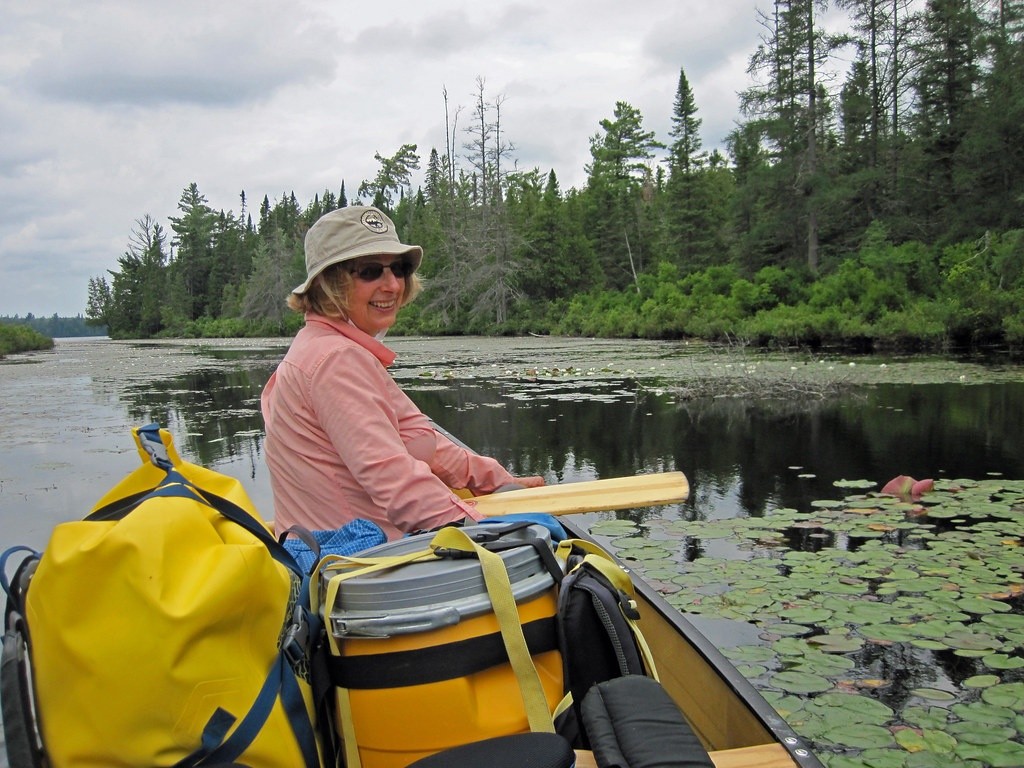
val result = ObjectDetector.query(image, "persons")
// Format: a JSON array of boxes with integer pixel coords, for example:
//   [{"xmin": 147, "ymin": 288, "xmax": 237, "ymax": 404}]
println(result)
[{"xmin": 261, "ymin": 207, "xmax": 544, "ymax": 544}]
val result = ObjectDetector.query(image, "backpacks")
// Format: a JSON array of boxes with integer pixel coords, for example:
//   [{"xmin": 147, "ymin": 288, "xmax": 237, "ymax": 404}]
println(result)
[{"xmin": 0, "ymin": 422, "xmax": 341, "ymax": 768}]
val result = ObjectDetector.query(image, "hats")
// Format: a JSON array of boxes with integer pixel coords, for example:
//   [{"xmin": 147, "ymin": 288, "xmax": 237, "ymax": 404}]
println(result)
[{"xmin": 292, "ymin": 205, "xmax": 424, "ymax": 295}]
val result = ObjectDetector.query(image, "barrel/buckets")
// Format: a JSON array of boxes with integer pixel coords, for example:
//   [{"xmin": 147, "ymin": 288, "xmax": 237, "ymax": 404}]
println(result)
[{"xmin": 318, "ymin": 524, "xmax": 564, "ymax": 768}]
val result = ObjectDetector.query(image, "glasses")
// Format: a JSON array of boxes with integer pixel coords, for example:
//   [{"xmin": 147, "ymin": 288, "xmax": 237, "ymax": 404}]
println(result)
[{"xmin": 354, "ymin": 258, "xmax": 415, "ymax": 282}]
